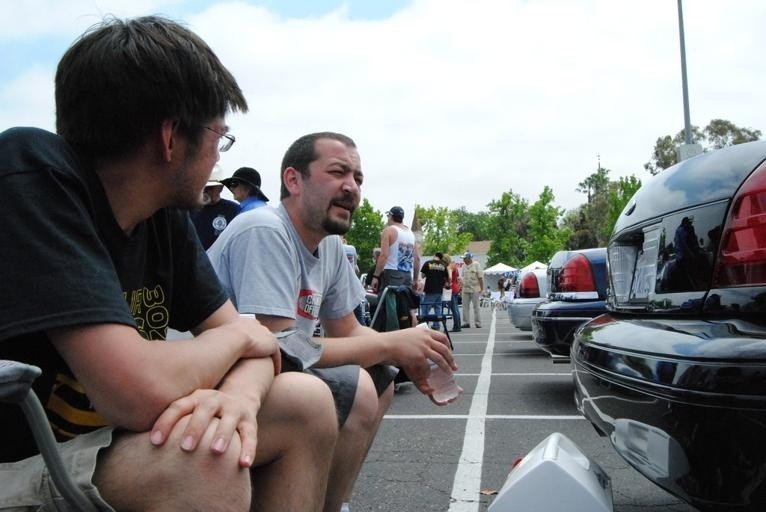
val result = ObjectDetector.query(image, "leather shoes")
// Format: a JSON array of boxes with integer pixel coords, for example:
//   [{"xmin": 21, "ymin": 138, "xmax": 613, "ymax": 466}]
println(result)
[{"xmin": 461, "ymin": 324, "xmax": 469, "ymax": 328}]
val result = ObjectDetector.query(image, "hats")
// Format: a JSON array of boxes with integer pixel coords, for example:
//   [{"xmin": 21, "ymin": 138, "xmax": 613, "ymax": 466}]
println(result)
[
  {"xmin": 385, "ymin": 206, "xmax": 403, "ymax": 217},
  {"xmin": 205, "ymin": 176, "xmax": 224, "ymax": 187},
  {"xmin": 220, "ymin": 168, "xmax": 269, "ymax": 201},
  {"xmin": 460, "ymin": 252, "xmax": 472, "ymax": 259}
]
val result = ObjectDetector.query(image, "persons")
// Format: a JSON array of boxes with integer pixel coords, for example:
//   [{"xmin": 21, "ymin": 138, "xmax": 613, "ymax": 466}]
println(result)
[
  {"xmin": 498, "ymin": 276, "xmax": 505, "ymax": 298},
  {"xmin": 204, "ymin": 131, "xmax": 460, "ymax": 512},
  {"xmin": 344, "ymin": 244, "xmax": 365, "ymax": 325},
  {"xmin": 196, "ymin": 175, "xmax": 238, "ymax": 251},
  {"xmin": 419, "ymin": 252, "xmax": 450, "ymax": 331},
  {"xmin": 371, "ymin": 206, "xmax": 421, "ymax": 302},
  {"xmin": 0, "ymin": 13, "xmax": 339, "ymax": 512},
  {"xmin": 365, "ymin": 247, "xmax": 381, "ymax": 285},
  {"xmin": 221, "ymin": 166, "xmax": 271, "ymax": 213},
  {"xmin": 442, "ymin": 254, "xmax": 462, "ymax": 334},
  {"xmin": 459, "ymin": 251, "xmax": 484, "ymax": 329}
]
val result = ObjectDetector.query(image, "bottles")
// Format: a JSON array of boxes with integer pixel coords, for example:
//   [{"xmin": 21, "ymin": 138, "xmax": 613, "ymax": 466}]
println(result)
[{"xmin": 416, "ymin": 322, "xmax": 458, "ymax": 403}]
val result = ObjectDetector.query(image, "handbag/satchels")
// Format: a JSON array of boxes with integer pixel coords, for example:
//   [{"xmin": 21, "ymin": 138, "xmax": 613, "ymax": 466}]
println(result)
[{"xmin": 441, "ymin": 288, "xmax": 452, "ymax": 302}]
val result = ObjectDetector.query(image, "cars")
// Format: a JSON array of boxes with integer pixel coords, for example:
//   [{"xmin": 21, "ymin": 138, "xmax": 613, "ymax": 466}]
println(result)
[
  {"xmin": 570, "ymin": 142, "xmax": 764, "ymax": 511},
  {"xmin": 507, "ymin": 248, "xmax": 609, "ymax": 364}
]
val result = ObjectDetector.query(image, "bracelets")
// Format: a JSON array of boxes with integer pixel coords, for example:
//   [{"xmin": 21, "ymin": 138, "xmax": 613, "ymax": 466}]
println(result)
[{"xmin": 372, "ymin": 275, "xmax": 379, "ymax": 279}]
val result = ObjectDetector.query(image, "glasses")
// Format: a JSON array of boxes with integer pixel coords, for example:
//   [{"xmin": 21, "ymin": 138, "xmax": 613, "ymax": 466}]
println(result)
[
  {"xmin": 386, "ymin": 215, "xmax": 388, "ymax": 217},
  {"xmin": 229, "ymin": 180, "xmax": 239, "ymax": 187},
  {"xmin": 205, "ymin": 127, "xmax": 236, "ymax": 152}
]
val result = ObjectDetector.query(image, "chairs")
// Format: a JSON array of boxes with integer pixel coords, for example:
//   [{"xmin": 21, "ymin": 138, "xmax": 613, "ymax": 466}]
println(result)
[
  {"xmin": 416, "ymin": 302, "xmax": 454, "ymax": 351},
  {"xmin": 0, "ymin": 358, "xmax": 99, "ymax": 512}
]
